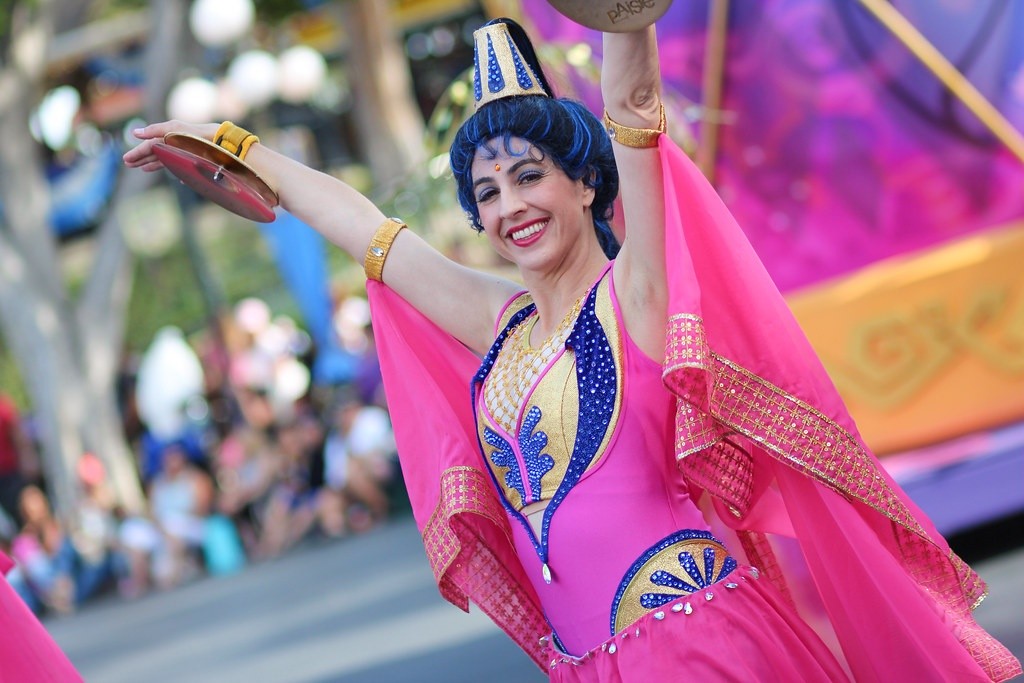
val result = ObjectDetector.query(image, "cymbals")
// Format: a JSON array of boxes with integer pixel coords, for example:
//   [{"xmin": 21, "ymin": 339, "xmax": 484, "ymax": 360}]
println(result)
[
  {"xmin": 146, "ymin": 131, "xmax": 279, "ymax": 226},
  {"xmin": 547, "ymin": 1, "xmax": 674, "ymax": 34}
]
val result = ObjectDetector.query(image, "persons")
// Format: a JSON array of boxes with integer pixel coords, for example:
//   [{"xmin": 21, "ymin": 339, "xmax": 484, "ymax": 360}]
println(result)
[
  {"xmin": 0, "ymin": 296, "xmax": 399, "ymax": 620},
  {"xmin": 122, "ymin": 1, "xmax": 1021, "ymax": 683}
]
